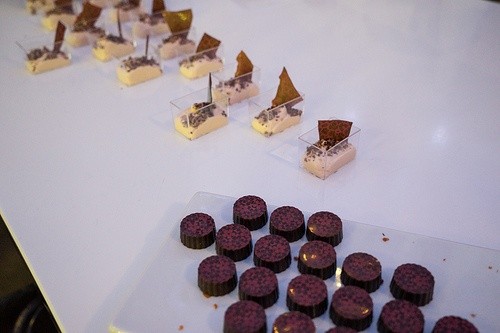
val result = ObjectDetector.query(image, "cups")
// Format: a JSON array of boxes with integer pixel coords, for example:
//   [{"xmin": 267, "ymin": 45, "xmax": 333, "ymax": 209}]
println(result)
[
  {"xmin": 208, "ymin": 60, "xmax": 261, "ymax": 105},
  {"xmin": 249, "ymin": 87, "xmax": 305, "ymax": 136},
  {"xmin": 167, "ymin": 86, "xmax": 230, "ymax": 141},
  {"xmin": 298, "ymin": 117, "xmax": 362, "ymax": 180},
  {"xmin": 23, "ymin": 0, "xmax": 164, "ymax": 86},
  {"xmin": 108, "ymin": 0, "xmax": 224, "ymax": 80}
]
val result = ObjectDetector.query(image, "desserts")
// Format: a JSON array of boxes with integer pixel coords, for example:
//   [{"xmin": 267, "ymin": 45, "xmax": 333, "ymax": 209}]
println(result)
[
  {"xmin": 211, "ymin": 50, "xmax": 260, "ymax": 103},
  {"xmin": 179, "ymin": 193, "xmax": 480, "ymax": 333},
  {"xmin": 253, "ymin": 66, "xmax": 303, "ymax": 137},
  {"xmin": 173, "ymin": 71, "xmax": 229, "ymax": 139},
  {"xmin": 302, "ymin": 117, "xmax": 356, "ymax": 178},
  {"xmin": 22, "ymin": 0, "xmax": 224, "ymax": 85}
]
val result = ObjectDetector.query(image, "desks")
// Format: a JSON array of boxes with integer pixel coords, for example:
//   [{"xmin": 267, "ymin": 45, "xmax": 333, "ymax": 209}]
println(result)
[{"xmin": 0, "ymin": 0, "xmax": 500, "ymax": 333}]
